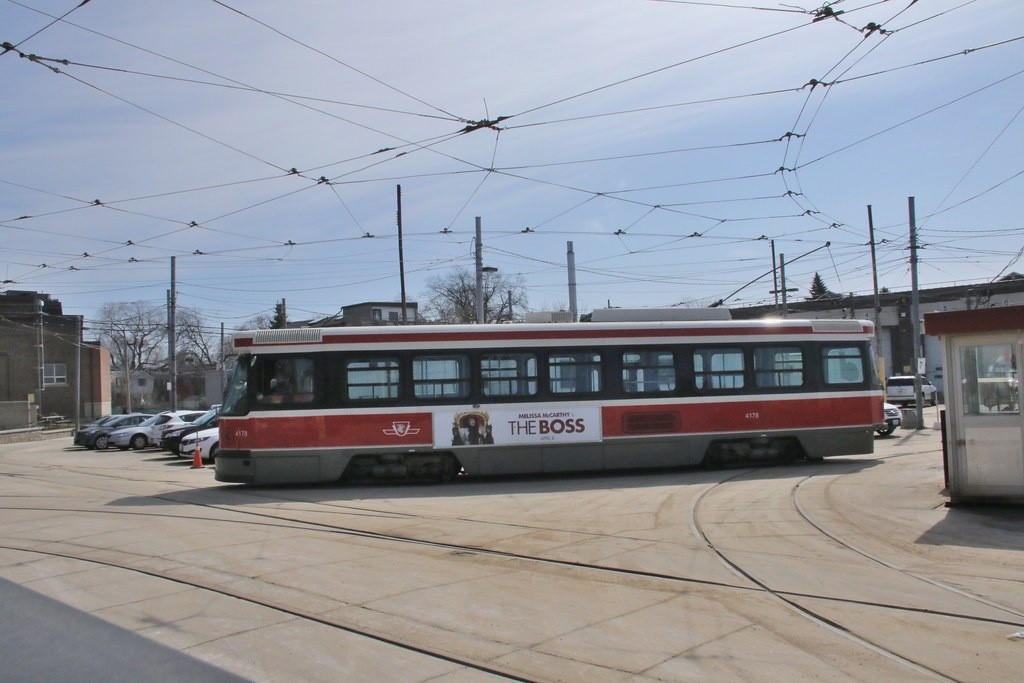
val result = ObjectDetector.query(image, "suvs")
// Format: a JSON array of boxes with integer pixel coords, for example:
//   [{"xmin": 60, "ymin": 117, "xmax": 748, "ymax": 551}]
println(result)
[{"xmin": 885, "ymin": 375, "xmax": 938, "ymax": 407}]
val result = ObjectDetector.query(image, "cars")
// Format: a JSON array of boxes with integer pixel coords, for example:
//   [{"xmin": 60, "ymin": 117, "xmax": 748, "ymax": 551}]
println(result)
[
  {"xmin": 874, "ymin": 403, "xmax": 903, "ymax": 436},
  {"xmin": 73, "ymin": 403, "xmax": 223, "ymax": 463}
]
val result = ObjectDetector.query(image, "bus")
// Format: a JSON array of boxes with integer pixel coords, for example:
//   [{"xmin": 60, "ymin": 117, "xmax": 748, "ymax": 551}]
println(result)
[{"xmin": 212, "ymin": 242, "xmax": 889, "ymax": 490}]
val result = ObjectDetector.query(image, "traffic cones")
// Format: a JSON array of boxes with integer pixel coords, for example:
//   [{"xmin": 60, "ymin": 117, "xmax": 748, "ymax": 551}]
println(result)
[{"xmin": 190, "ymin": 443, "xmax": 205, "ymax": 468}]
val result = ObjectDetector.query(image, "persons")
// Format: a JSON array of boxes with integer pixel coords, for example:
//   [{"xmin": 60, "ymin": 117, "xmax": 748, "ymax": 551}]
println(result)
[{"xmin": 256, "ymin": 373, "xmax": 292, "ymax": 400}]
